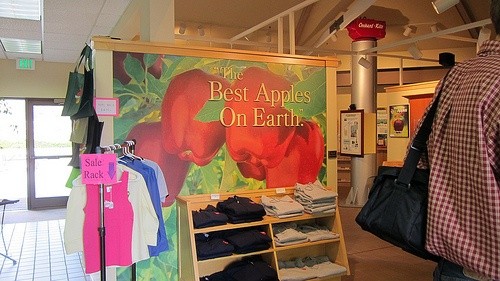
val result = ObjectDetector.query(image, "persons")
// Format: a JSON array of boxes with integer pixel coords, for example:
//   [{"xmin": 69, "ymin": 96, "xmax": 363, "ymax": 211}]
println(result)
[{"xmin": 402, "ymin": 0, "xmax": 499, "ymax": 281}]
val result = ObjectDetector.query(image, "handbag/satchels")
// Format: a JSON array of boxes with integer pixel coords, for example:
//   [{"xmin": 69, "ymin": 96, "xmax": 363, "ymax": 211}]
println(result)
[
  {"xmin": 61, "ymin": 45, "xmax": 105, "ymax": 169},
  {"xmin": 355, "ymin": 161, "xmax": 442, "ymax": 263}
]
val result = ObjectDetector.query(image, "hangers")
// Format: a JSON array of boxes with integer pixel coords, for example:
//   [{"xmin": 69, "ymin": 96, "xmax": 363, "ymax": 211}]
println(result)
[{"xmin": 80, "ymin": 141, "xmax": 144, "ymax": 184}]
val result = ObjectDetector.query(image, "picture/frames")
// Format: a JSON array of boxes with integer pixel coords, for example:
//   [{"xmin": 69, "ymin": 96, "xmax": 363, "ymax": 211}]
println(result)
[
  {"xmin": 388, "ymin": 103, "xmax": 410, "ymax": 138},
  {"xmin": 338, "ymin": 109, "xmax": 365, "ymax": 158}
]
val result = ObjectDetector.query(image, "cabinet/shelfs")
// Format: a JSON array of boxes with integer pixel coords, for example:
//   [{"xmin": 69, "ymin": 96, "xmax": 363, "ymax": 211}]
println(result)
[{"xmin": 178, "ymin": 187, "xmax": 351, "ymax": 281}]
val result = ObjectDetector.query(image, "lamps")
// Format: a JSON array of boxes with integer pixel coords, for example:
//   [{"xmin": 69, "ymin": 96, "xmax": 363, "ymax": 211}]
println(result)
[
  {"xmin": 178, "ymin": 22, "xmax": 187, "ymax": 34},
  {"xmin": 198, "ymin": 24, "xmax": 208, "ymax": 36},
  {"xmin": 244, "ymin": 0, "xmax": 493, "ymax": 70}
]
